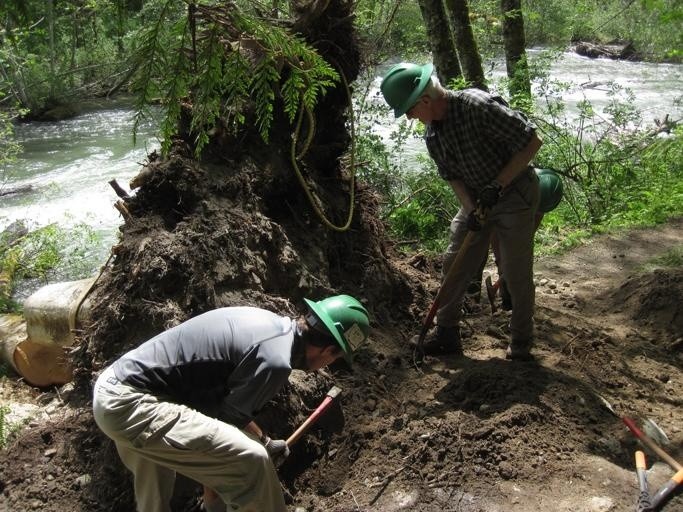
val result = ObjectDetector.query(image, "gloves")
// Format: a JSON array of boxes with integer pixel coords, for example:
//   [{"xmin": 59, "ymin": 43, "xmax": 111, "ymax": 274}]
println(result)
[
  {"xmin": 263, "ymin": 436, "xmax": 291, "ymax": 468},
  {"xmin": 466, "ymin": 182, "xmax": 504, "ymax": 232}
]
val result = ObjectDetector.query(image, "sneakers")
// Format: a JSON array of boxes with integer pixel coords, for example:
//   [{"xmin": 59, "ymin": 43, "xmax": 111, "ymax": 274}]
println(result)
[
  {"xmin": 506, "ymin": 340, "xmax": 533, "ymax": 359},
  {"xmin": 409, "ymin": 325, "xmax": 460, "ymax": 355}
]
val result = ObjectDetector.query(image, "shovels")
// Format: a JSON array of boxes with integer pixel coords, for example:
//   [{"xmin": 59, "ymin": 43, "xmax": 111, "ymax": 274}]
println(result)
[
  {"xmin": 403, "ymin": 230, "xmax": 476, "ymax": 369},
  {"xmin": 589, "ymin": 386, "xmax": 683, "ymax": 473}
]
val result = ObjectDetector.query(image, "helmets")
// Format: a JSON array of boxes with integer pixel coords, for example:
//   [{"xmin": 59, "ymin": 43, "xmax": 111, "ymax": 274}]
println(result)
[
  {"xmin": 536, "ymin": 170, "xmax": 563, "ymax": 212},
  {"xmin": 380, "ymin": 62, "xmax": 434, "ymax": 119},
  {"xmin": 304, "ymin": 293, "xmax": 371, "ymax": 364}
]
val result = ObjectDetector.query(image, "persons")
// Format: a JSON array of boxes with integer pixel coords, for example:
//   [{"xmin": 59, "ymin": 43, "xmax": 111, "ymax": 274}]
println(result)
[
  {"xmin": 441, "ymin": 167, "xmax": 564, "ymax": 313},
  {"xmin": 89, "ymin": 292, "xmax": 371, "ymax": 511},
  {"xmin": 378, "ymin": 61, "xmax": 543, "ymax": 363}
]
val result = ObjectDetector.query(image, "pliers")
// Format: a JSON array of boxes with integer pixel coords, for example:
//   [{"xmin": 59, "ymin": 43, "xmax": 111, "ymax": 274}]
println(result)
[{"xmin": 635, "ymin": 451, "xmax": 683, "ymax": 512}]
